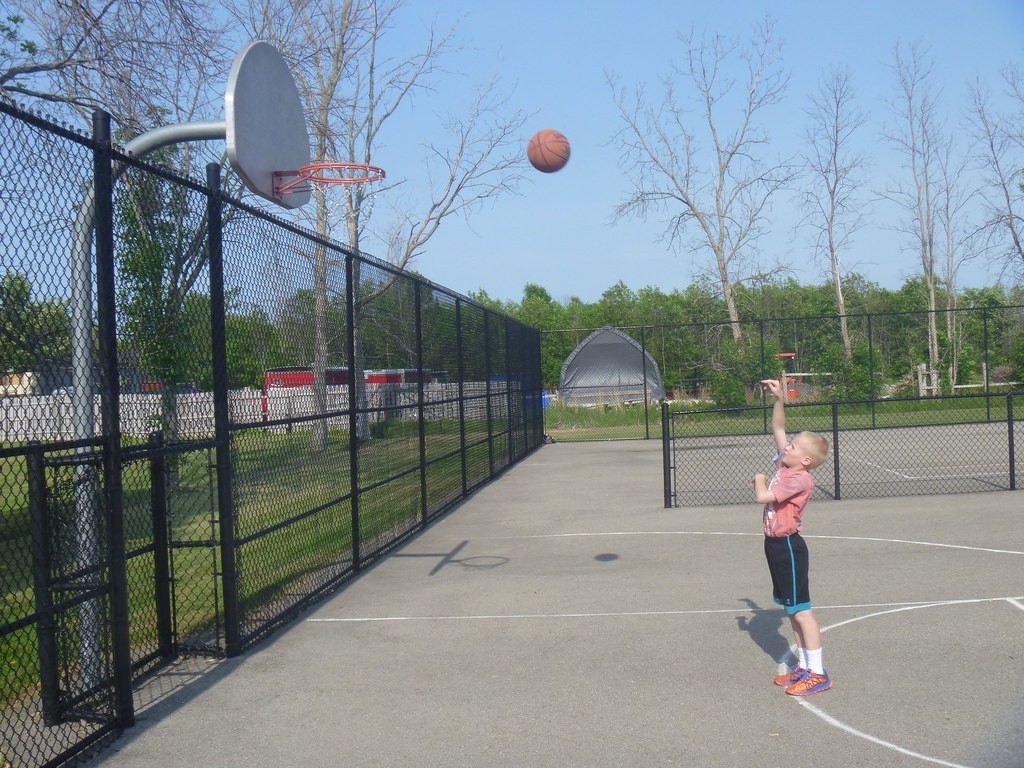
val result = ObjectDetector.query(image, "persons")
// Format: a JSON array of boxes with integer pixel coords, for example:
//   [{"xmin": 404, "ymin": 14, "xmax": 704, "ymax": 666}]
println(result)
[{"xmin": 750, "ymin": 378, "xmax": 833, "ymax": 696}]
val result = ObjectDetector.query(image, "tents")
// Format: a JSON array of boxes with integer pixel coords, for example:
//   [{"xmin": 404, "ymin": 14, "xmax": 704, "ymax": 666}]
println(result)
[{"xmin": 558, "ymin": 325, "xmax": 666, "ymax": 412}]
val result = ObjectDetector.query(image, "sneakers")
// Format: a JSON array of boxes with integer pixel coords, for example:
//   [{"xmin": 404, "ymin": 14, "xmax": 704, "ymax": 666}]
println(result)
[
  {"xmin": 775, "ymin": 666, "xmax": 805, "ymax": 685},
  {"xmin": 786, "ymin": 669, "xmax": 830, "ymax": 696}
]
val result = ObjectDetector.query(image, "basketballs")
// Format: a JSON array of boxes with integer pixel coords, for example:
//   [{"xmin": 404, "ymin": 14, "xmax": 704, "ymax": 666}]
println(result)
[{"xmin": 527, "ymin": 129, "xmax": 571, "ymax": 173}]
